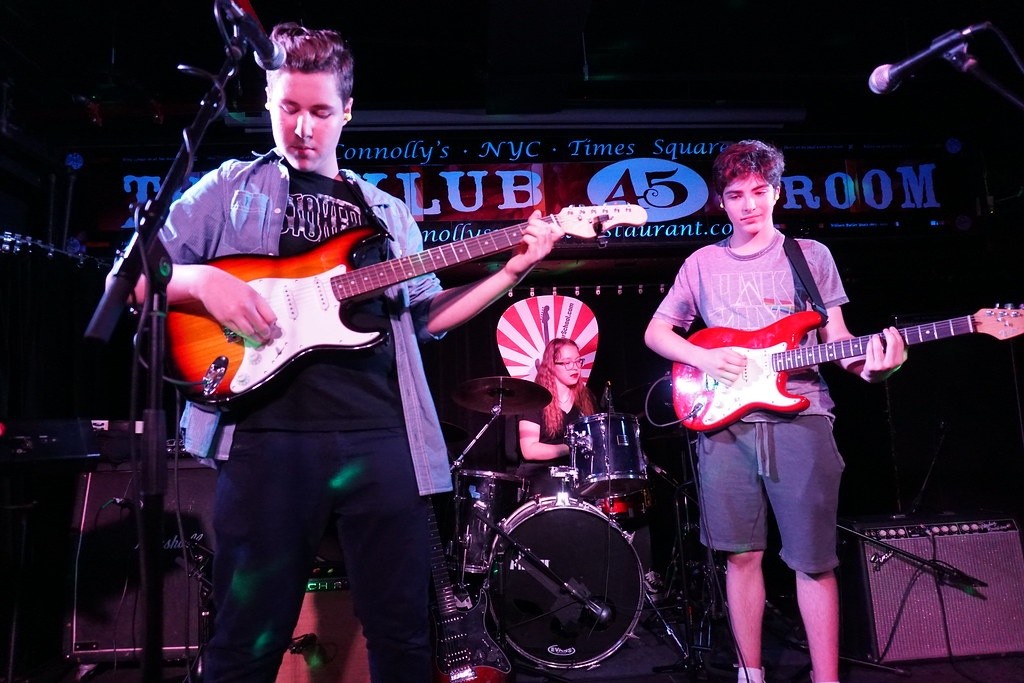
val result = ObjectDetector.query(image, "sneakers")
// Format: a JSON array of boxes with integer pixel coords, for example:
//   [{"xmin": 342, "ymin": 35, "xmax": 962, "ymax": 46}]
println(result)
[{"xmin": 643, "ymin": 567, "xmax": 665, "ymax": 593}]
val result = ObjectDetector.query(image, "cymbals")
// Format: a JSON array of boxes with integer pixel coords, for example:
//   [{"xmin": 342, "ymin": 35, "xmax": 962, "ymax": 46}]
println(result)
[{"xmin": 444, "ymin": 375, "xmax": 552, "ymax": 417}]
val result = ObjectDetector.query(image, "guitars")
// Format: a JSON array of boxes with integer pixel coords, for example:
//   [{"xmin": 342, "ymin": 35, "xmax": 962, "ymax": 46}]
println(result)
[
  {"xmin": 670, "ymin": 303, "xmax": 1024, "ymax": 434},
  {"xmin": 166, "ymin": 201, "xmax": 650, "ymax": 414},
  {"xmin": 419, "ymin": 494, "xmax": 511, "ymax": 677}
]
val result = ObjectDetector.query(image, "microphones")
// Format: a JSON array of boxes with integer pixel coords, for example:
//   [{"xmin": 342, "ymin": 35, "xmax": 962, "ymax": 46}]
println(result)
[
  {"xmin": 601, "ymin": 383, "xmax": 611, "ymax": 408},
  {"xmin": 219, "ymin": 0, "xmax": 282, "ymax": 68},
  {"xmin": 113, "ymin": 496, "xmax": 144, "ymax": 513},
  {"xmin": 869, "ymin": 23, "xmax": 991, "ymax": 94},
  {"xmin": 589, "ymin": 601, "xmax": 613, "ymax": 623},
  {"xmin": 942, "ymin": 572, "xmax": 987, "ymax": 587},
  {"xmin": 291, "ymin": 633, "xmax": 316, "ymax": 654}
]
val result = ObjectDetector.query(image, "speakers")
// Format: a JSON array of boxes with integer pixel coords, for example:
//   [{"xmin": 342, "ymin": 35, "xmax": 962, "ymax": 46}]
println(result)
[
  {"xmin": 274, "ymin": 577, "xmax": 372, "ymax": 683},
  {"xmin": 61, "ymin": 457, "xmax": 219, "ymax": 663},
  {"xmin": 838, "ymin": 518, "xmax": 1024, "ymax": 667}
]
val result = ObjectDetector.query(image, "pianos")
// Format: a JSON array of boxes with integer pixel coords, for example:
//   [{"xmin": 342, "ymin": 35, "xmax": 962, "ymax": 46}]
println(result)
[{"xmin": 4, "ymin": 417, "xmax": 152, "ymax": 467}]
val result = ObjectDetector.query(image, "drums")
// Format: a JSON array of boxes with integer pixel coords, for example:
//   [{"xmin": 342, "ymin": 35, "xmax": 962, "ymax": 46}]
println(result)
[
  {"xmin": 486, "ymin": 496, "xmax": 647, "ymax": 669},
  {"xmin": 446, "ymin": 467, "xmax": 533, "ymax": 575},
  {"xmin": 561, "ymin": 410, "xmax": 652, "ymax": 484}
]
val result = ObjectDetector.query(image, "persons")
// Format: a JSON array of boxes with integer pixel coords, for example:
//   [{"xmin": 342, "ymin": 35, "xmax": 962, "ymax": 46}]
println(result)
[
  {"xmin": 515, "ymin": 339, "xmax": 676, "ymax": 602},
  {"xmin": 643, "ymin": 140, "xmax": 907, "ymax": 683},
  {"xmin": 121, "ymin": 23, "xmax": 566, "ymax": 683}
]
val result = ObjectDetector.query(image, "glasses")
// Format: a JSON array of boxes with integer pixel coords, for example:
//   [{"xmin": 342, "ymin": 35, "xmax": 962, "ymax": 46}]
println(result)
[{"xmin": 554, "ymin": 359, "xmax": 584, "ymax": 371}]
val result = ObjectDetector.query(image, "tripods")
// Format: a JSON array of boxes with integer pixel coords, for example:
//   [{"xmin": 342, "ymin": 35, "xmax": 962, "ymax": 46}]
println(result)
[{"xmin": 651, "ymin": 432, "xmax": 807, "ymax": 683}]
